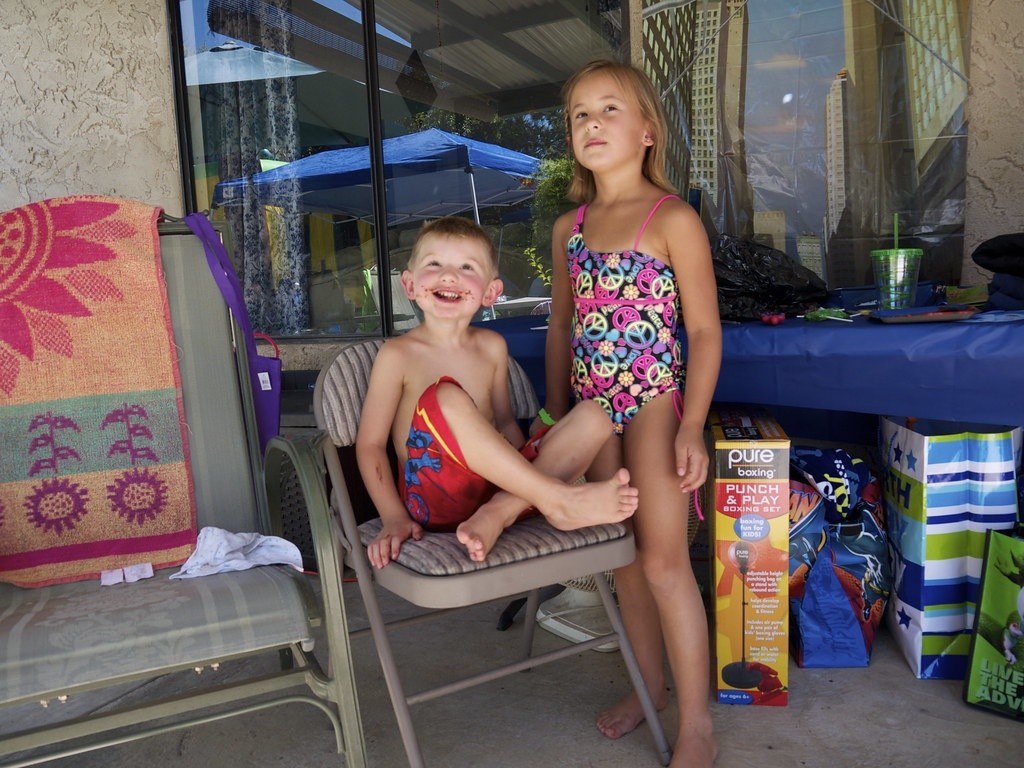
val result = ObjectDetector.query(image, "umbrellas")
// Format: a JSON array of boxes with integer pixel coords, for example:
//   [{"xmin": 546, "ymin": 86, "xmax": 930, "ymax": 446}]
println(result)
[{"xmin": 183, "ymin": 42, "xmax": 433, "ymax": 168}]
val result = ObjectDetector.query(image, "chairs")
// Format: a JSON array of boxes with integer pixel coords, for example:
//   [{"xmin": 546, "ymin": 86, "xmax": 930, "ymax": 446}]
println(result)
[
  {"xmin": 0, "ymin": 197, "xmax": 367, "ymax": 768},
  {"xmin": 310, "ymin": 336, "xmax": 676, "ymax": 768}
]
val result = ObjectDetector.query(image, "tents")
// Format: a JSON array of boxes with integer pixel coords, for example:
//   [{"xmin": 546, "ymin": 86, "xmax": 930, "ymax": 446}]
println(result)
[{"xmin": 212, "ymin": 127, "xmax": 553, "ymax": 323}]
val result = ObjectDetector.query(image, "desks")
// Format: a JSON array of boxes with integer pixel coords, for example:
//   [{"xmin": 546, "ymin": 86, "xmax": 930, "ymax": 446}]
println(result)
[{"xmin": 470, "ymin": 311, "xmax": 1024, "ymax": 632}]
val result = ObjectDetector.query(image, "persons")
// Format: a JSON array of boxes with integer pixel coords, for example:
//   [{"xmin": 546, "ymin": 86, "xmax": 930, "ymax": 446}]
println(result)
[
  {"xmin": 540, "ymin": 60, "xmax": 725, "ymax": 768},
  {"xmin": 354, "ymin": 214, "xmax": 641, "ymax": 570}
]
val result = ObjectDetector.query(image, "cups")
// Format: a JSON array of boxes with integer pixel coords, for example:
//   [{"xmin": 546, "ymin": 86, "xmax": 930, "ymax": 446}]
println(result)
[{"xmin": 871, "ymin": 248, "xmax": 924, "ymax": 309}]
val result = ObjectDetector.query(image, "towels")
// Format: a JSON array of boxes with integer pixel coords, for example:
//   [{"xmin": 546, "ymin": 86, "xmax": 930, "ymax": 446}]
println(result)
[
  {"xmin": 0, "ymin": 193, "xmax": 203, "ymax": 589},
  {"xmin": 169, "ymin": 525, "xmax": 305, "ymax": 580}
]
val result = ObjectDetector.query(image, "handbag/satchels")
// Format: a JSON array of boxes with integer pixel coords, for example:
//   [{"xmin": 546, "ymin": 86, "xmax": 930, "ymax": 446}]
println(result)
[
  {"xmin": 713, "ymin": 230, "xmax": 828, "ymax": 322},
  {"xmin": 884, "ymin": 413, "xmax": 1021, "ymax": 680},
  {"xmin": 786, "ymin": 441, "xmax": 891, "ymax": 669},
  {"xmin": 964, "ymin": 524, "xmax": 1024, "ymax": 724}
]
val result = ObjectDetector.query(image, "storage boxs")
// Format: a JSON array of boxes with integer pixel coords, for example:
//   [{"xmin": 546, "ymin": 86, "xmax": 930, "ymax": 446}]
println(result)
[{"xmin": 706, "ymin": 405, "xmax": 793, "ymax": 707}]
